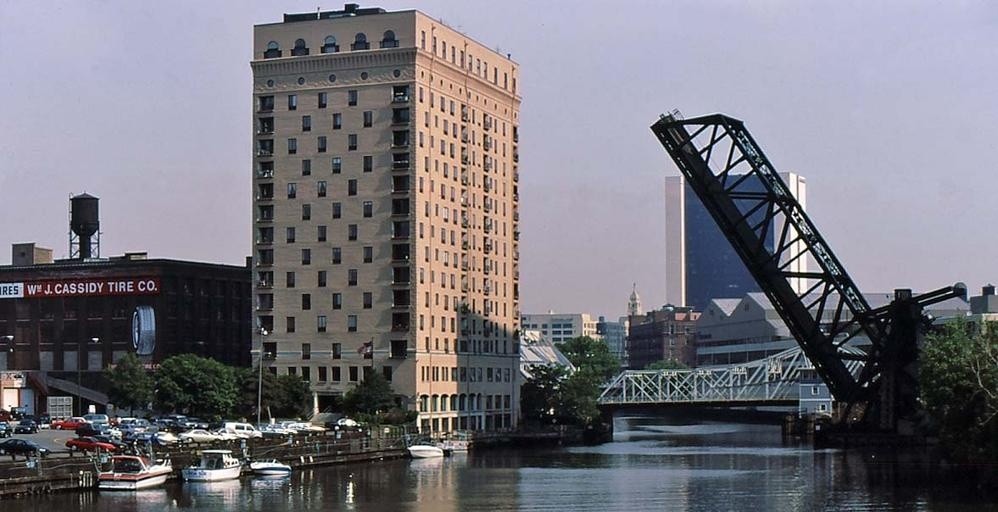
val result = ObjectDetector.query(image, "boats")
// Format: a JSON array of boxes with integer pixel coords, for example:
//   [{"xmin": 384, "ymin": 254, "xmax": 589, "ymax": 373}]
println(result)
[
  {"xmin": 407, "ymin": 445, "xmax": 444, "ymax": 459},
  {"xmin": 93, "ymin": 450, "xmax": 293, "ymax": 490}
]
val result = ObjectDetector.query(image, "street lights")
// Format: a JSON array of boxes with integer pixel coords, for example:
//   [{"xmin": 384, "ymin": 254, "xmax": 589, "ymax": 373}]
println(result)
[
  {"xmin": 250, "ymin": 327, "xmax": 272, "ymax": 431},
  {"xmin": 0, "ymin": 335, "xmax": 14, "ymax": 343},
  {"xmin": 79, "ymin": 336, "xmax": 100, "ymax": 416}
]
